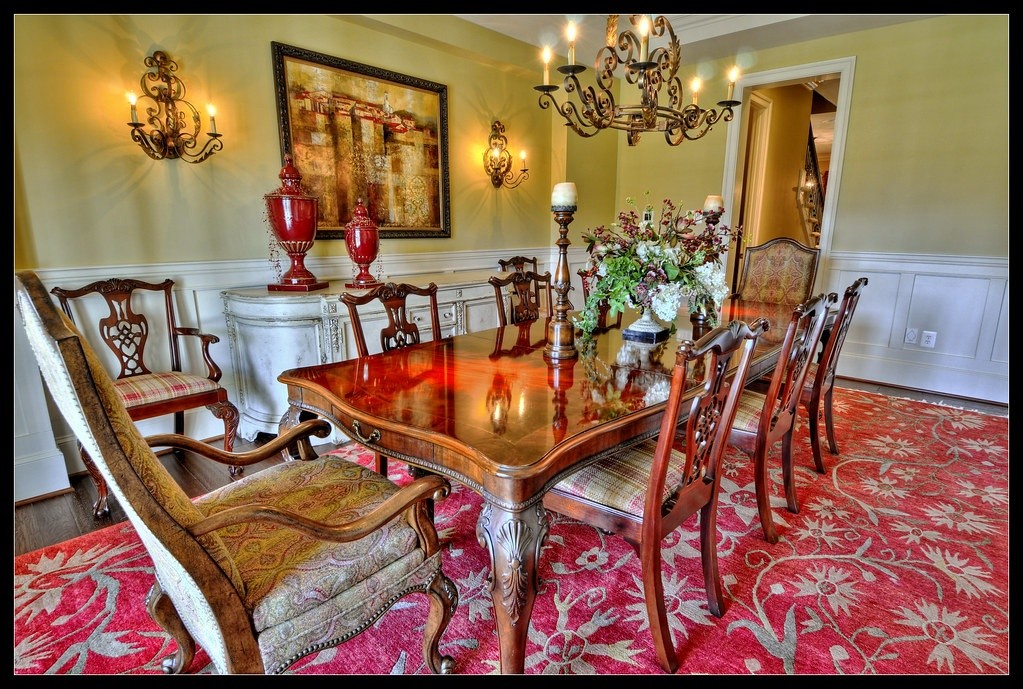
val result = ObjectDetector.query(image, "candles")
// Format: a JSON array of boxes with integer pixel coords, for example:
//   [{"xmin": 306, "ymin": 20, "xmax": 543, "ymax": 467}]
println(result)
[
  {"xmin": 550, "ymin": 181, "xmax": 577, "ymax": 211},
  {"xmin": 703, "ymin": 196, "xmax": 723, "ymax": 215}
]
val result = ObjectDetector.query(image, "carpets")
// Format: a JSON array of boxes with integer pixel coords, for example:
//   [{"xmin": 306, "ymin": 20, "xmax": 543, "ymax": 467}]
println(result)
[{"xmin": 13, "ymin": 386, "xmax": 1009, "ymax": 674}]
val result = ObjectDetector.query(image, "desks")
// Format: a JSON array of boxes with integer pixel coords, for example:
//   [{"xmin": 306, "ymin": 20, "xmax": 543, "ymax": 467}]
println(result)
[{"xmin": 278, "ymin": 300, "xmax": 839, "ymax": 674}]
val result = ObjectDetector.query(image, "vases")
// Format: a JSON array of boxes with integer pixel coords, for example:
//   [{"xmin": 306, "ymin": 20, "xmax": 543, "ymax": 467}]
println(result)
[
  {"xmin": 345, "ymin": 198, "xmax": 386, "ymax": 289},
  {"xmin": 264, "ymin": 154, "xmax": 329, "ymax": 292},
  {"xmin": 622, "ymin": 306, "xmax": 670, "ymax": 339}
]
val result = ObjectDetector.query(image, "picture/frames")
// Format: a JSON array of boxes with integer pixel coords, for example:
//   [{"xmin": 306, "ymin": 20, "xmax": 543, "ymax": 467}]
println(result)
[{"xmin": 271, "ymin": 41, "xmax": 451, "ymax": 240}]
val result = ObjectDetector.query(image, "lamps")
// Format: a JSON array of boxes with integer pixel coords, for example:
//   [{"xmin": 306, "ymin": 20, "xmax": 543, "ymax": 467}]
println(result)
[
  {"xmin": 483, "ymin": 120, "xmax": 530, "ymax": 190},
  {"xmin": 126, "ymin": 49, "xmax": 222, "ymax": 164},
  {"xmin": 533, "ymin": 15, "xmax": 741, "ymax": 147}
]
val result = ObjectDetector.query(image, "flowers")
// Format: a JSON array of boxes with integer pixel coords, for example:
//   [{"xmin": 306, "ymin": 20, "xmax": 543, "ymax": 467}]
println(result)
[{"xmin": 576, "ymin": 191, "xmax": 752, "ymax": 382}]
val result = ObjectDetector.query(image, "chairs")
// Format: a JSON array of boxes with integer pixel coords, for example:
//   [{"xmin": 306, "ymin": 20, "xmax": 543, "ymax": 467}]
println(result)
[{"xmin": 14, "ymin": 235, "xmax": 871, "ymax": 674}]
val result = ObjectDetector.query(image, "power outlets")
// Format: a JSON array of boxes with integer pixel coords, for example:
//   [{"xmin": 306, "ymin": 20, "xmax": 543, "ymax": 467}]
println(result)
[{"xmin": 921, "ymin": 331, "xmax": 937, "ymax": 348}]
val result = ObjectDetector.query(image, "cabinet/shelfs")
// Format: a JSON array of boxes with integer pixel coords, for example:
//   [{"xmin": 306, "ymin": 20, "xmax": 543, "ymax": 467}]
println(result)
[{"xmin": 219, "ymin": 268, "xmax": 515, "ymax": 447}]
